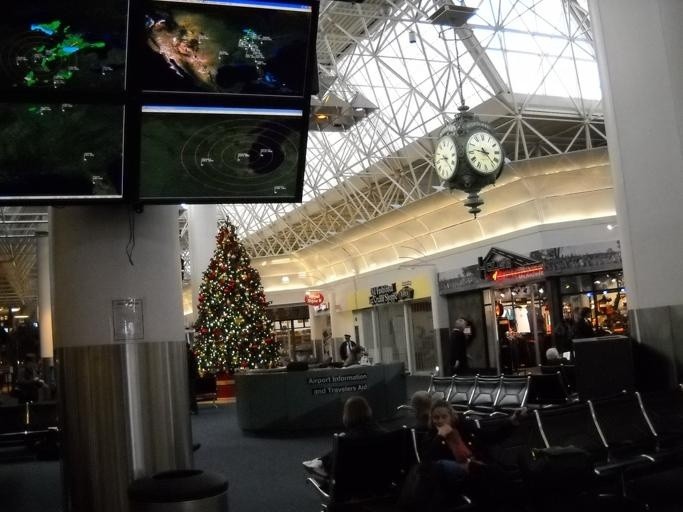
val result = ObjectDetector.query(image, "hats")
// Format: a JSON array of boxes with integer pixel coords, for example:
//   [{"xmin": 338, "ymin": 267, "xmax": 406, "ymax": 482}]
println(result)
[{"xmin": 344, "ymin": 335, "xmax": 351, "ymax": 338}]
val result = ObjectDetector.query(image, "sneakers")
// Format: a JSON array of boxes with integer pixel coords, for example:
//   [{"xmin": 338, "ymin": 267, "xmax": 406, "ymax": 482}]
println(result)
[{"xmin": 303, "ymin": 458, "xmax": 323, "ymax": 474}]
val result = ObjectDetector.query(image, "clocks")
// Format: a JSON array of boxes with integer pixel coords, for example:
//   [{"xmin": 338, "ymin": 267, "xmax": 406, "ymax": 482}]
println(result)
[
  {"xmin": 467, "ymin": 132, "xmax": 502, "ymax": 175},
  {"xmin": 434, "ymin": 136, "xmax": 458, "ymax": 181}
]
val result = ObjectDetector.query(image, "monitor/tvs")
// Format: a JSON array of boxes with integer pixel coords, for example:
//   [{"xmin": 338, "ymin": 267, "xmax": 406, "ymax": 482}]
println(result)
[
  {"xmin": 2, "ymin": 94, "xmax": 132, "ymax": 204},
  {"xmin": 135, "ymin": 1, "xmax": 321, "ymax": 97},
  {"xmin": 136, "ymin": 97, "xmax": 310, "ymax": 203},
  {"xmin": 0, "ymin": 0, "xmax": 131, "ymax": 95}
]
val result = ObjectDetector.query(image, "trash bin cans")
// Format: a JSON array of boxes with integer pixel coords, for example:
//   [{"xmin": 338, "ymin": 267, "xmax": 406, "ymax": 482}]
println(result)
[{"xmin": 127, "ymin": 468, "xmax": 228, "ymax": 512}]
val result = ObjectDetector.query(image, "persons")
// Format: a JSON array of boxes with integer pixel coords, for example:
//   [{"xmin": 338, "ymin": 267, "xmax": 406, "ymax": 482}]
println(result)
[
  {"xmin": 301, "ymin": 396, "xmax": 390, "ymax": 491},
  {"xmin": 12, "ymin": 366, "xmax": 43, "ymax": 403},
  {"xmin": 18, "ymin": 361, "xmax": 50, "ymax": 403},
  {"xmin": 426, "ymin": 398, "xmax": 505, "ymax": 507},
  {"xmin": 409, "ymin": 391, "xmax": 435, "ymax": 429},
  {"xmin": 185, "ymin": 341, "xmax": 201, "ymax": 414},
  {"xmin": 446, "ymin": 318, "xmax": 477, "ymax": 372},
  {"xmin": 573, "ymin": 306, "xmax": 596, "ymax": 336},
  {"xmin": 541, "ymin": 346, "xmax": 572, "ymax": 366},
  {"xmin": 337, "ymin": 331, "xmax": 359, "ymax": 364}
]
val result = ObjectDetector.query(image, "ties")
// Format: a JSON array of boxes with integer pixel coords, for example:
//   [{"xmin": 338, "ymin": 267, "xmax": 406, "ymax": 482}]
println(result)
[{"xmin": 349, "ymin": 344, "xmax": 352, "ymax": 352}]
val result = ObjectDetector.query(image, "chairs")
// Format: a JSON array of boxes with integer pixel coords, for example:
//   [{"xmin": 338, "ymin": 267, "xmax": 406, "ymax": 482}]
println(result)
[
  {"xmin": 1, "ymin": 400, "xmax": 61, "ymax": 459},
  {"xmin": 194, "ymin": 377, "xmax": 218, "ymax": 409}
]
